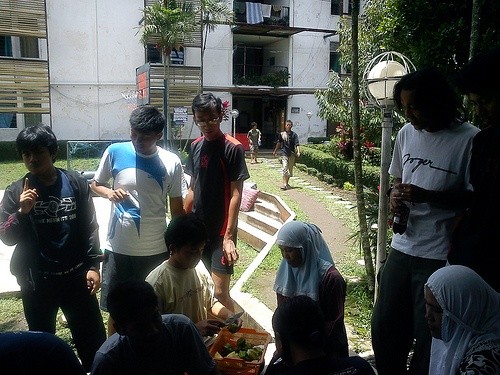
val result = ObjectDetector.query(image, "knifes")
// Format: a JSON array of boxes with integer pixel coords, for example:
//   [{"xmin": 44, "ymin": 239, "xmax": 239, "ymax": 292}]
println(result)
[{"xmin": 209, "ymin": 311, "xmax": 244, "ymax": 336}]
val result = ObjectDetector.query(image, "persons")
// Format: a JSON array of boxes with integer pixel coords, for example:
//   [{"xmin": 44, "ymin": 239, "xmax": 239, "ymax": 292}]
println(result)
[
  {"xmin": 273, "ymin": 120, "xmax": 300, "ymax": 189},
  {"xmin": 264, "ymin": 296, "xmax": 377, "ymax": 375},
  {"xmin": 91, "ymin": 282, "xmax": 222, "ymax": 375},
  {"xmin": 247, "ymin": 122, "xmax": 261, "ymax": 163},
  {"xmin": 425, "ymin": 265, "xmax": 500, "ymax": 375},
  {"xmin": 90, "ymin": 105, "xmax": 185, "ymax": 346},
  {"xmin": 273, "ymin": 222, "xmax": 348, "ymax": 361},
  {"xmin": 183, "ymin": 92, "xmax": 249, "ymax": 317},
  {"xmin": 465, "ymin": 64, "xmax": 500, "ymax": 291},
  {"xmin": 0, "ymin": 124, "xmax": 107, "ymax": 372},
  {"xmin": 371, "ymin": 66, "xmax": 480, "ymax": 375},
  {"xmin": 145, "ymin": 217, "xmax": 243, "ymax": 334}
]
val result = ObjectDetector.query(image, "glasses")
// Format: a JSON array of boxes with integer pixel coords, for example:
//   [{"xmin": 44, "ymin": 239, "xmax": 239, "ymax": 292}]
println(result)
[{"xmin": 195, "ymin": 117, "xmax": 220, "ymax": 127}]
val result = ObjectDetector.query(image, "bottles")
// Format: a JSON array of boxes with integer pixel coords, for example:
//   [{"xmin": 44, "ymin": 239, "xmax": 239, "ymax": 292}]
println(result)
[{"xmin": 391, "ymin": 184, "xmax": 410, "ymax": 234}]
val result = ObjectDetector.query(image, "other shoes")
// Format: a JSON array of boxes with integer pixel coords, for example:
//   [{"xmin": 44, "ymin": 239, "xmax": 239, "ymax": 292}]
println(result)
[
  {"xmin": 280, "ymin": 184, "xmax": 291, "ymax": 190},
  {"xmin": 250, "ymin": 160, "xmax": 257, "ymax": 164}
]
